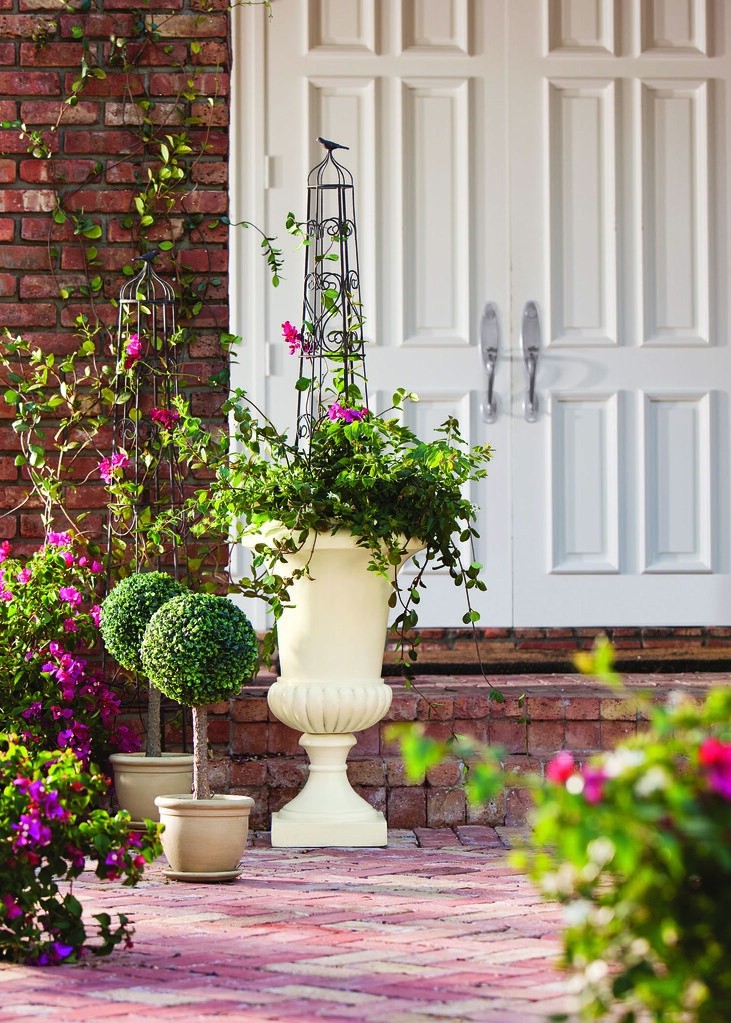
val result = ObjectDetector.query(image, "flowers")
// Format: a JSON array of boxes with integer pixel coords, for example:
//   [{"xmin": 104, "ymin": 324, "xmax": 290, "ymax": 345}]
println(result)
[
  {"xmin": 0, "ymin": 524, "xmax": 168, "ymax": 970},
  {"xmin": 386, "ymin": 631, "xmax": 731, "ymax": 1023},
  {"xmin": 94, "ymin": 319, "xmax": 534, "ymax": 767}
]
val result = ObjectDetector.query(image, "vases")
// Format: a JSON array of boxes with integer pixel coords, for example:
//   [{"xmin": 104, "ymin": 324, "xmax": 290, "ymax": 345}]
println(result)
[{"xmin": 230, "ymin": 516, "xmax": 428, "ymax": 851}]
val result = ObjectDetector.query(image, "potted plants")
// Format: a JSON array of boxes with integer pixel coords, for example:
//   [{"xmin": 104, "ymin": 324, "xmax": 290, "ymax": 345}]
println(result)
[
  {"xmin": 142, "ymin": 589, "xmax": 263, "ymax": 888},
  {"xmin": 95, "ymin": 569, "xmax": 197, "ymax": 829}
]
